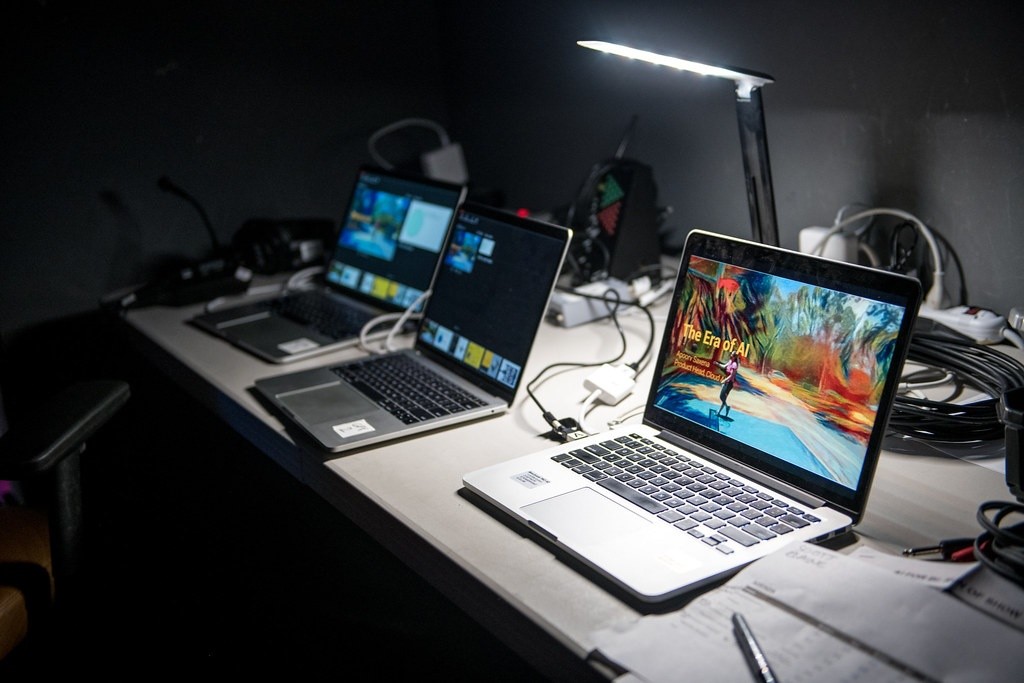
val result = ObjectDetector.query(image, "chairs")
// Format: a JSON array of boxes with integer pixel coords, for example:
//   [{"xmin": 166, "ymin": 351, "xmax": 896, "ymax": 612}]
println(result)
[{"xmin": 1, "ymin": 381, "xmax": 129, "ymax": 682}]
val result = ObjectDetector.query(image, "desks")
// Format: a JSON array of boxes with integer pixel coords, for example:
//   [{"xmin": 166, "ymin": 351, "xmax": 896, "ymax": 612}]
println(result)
[{"xmin": 106, "ymin": 264, "xmax": 1024, "ymax": 683}]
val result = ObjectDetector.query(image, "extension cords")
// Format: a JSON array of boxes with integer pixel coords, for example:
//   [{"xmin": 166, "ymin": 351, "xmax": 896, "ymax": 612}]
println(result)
[{"xmin": 917, "ymin": 300, "xmax": 1008, "ymax": 346}]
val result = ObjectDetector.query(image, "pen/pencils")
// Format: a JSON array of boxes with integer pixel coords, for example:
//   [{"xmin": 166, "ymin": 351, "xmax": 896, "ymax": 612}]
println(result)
[{"xmin": 732, "ymin": 612, "xmax": 779, "ymax": 682}]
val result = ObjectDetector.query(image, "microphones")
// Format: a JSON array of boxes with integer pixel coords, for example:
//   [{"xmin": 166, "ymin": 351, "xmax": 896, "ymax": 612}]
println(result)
[{"xmin": 157, "ymin": 174, "xmax": 226, "ymax": 259}]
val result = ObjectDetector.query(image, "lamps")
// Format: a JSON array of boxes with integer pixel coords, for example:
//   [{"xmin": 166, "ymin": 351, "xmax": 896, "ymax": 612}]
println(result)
[{"xmin": 577, "ymin": 37, "xmax": 780, "ymax": 246}]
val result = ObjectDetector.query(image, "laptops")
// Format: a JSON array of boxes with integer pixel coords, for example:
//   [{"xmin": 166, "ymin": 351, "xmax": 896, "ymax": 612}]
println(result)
[{"xmin": 191, "ymin": 159, "xmax": 923, "ymax": 604}]
